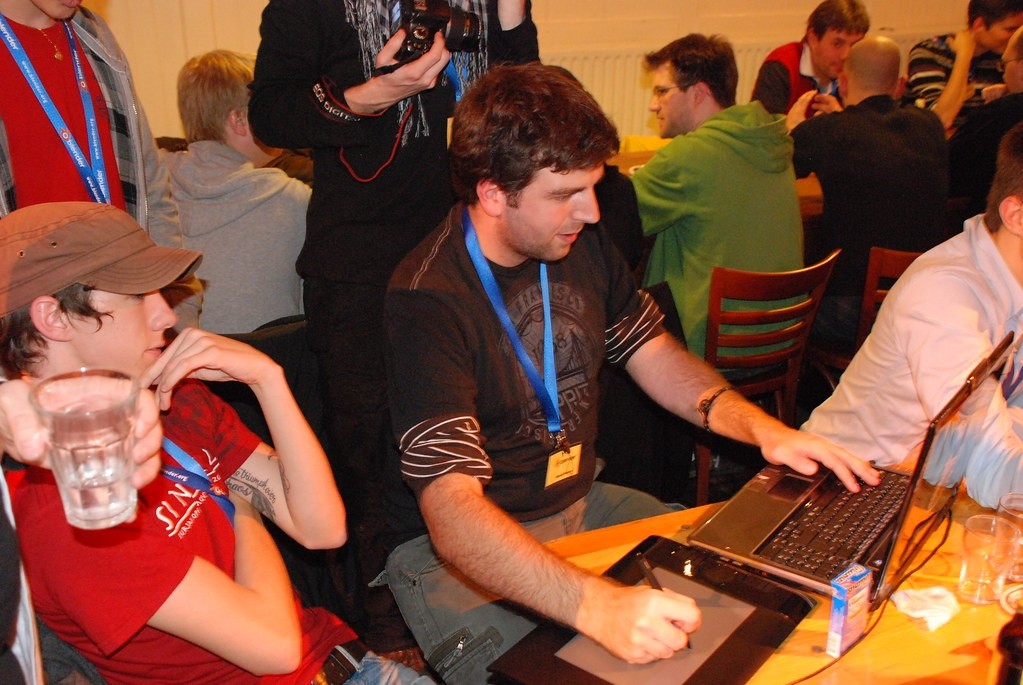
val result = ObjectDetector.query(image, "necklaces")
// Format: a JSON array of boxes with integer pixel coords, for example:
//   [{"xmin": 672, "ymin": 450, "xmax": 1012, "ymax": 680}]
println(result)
[{"xmin": 36, "ymin": 25, "xmax": 62, "ymax": 60}]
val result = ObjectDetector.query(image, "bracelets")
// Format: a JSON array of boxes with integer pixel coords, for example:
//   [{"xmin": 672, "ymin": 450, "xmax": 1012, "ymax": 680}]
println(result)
[{"xmin": 700, "ymin": 384, "xmax": 737, "ymax": 434}]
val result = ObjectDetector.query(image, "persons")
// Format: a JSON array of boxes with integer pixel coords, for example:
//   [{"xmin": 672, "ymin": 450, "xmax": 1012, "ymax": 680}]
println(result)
[
  {"xmin": 629, "ymin": 32, "xmax": 806, "ymax": 380},
  {"xmin": 250, "ymin": 0, "xmax": 539, "ymax": 649},
  {"xmin": 800, "ymin": 122, "xmax": 1023, "ymax": 510},
  {"xmin": 384, "ymin": 61, "xmax": 880, "ymax": 685},
  {"xmin": 0, "ymin": 369, "xmax": 163, "ymax": 685},
  {"xmin": 750, "ymin": 2, "xmax": 870, "ymax": 134},
  {"xmin": 0, "ymin": 201, "xmax": 438, "ymax": 685},
  {"xmin": 0, "ymin": 1, "xmax": 204, "ymax": 341},
  {"xmin": 791, "ymin": 35, "xmax": 950, "ymax": 413},
  {"xmin": 905, "ymin": 0, "xmax": 1022, "ymax": 227},
  {"xmin": 152, "ymin": 49, "xmax": 311, "ymax": 335}
]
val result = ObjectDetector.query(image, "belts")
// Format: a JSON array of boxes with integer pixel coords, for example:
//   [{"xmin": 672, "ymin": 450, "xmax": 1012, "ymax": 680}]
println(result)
[{"xmin": 311, "ymin": 638, "xmax": 370, "ymax": 685}]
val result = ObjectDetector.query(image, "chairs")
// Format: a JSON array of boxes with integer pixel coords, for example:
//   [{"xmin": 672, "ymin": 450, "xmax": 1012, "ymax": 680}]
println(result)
[
  {"xmin": 806, "ymin": 245, "xmax": 922, "ymax": 374},
  {"xmin": 687, "ymin": 248, "xmax": 841, "ymax": 501},
  {"xmin": 201, "ymin": 315, "xmax": 325, "ymax": 448}
]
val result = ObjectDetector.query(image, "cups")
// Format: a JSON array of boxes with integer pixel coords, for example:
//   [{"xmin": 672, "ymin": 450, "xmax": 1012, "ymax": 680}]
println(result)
[
  {"xmin": 28, "ymin": 367, "xmax": 141, "ymax": 530},
  {"xmin": 957, "ymin": 514, "xmax": 1021, "ymax": 605},
  {"xmin": 989, "ymin": 493, "xmax": 1023, "ymax": 583}
]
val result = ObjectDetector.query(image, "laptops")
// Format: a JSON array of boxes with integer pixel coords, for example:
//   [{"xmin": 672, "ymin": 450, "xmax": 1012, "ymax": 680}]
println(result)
[{"xmin": 688, "ymin": 330, "xmax": 1014, "ymax": 614}]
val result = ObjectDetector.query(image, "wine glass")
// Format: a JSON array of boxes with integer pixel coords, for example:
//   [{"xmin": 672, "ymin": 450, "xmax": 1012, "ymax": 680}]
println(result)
[{"xmin": 1000, "ymin": 585, "xmax": 1023, "ymax": 615}]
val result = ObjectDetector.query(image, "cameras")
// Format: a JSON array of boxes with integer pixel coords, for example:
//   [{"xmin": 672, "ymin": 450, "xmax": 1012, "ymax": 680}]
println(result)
[{"xmin": 386, "ymin": 0, "xmax": 481, "ymax": 63}]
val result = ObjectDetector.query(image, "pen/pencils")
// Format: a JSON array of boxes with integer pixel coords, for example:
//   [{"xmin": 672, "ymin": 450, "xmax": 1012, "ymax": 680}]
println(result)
[{"xmin": 635, "ymin": 552, "xmax": 693, "ymax": 650}]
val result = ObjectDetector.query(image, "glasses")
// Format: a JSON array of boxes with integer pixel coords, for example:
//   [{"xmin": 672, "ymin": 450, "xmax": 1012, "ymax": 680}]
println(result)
[{"xmin": 653, "ymin": 83, "xmax": 694, "ymax": 99}]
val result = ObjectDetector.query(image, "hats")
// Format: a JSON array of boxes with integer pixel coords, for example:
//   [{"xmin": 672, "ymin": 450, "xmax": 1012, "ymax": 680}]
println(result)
[{"xmin": 0, "ymin": 202, "xmax": 204, "ymax": 320}]
